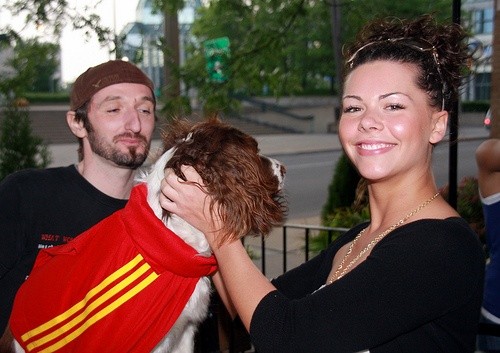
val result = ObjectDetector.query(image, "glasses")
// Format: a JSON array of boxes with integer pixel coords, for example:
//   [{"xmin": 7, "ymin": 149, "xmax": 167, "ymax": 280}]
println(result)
[{"xmin": 344, "ymin": 35, "xmax": 444, "ymax": 81}]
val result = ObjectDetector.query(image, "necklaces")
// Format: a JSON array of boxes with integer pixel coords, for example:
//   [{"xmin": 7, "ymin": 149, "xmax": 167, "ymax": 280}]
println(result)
[{"xmin": 312, "ymin": 192, "xmax": 440, "ymax": 293}]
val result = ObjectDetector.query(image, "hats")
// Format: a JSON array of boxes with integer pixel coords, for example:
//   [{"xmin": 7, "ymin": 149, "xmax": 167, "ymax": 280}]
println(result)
[{"xmin": 68, "ymin": 59, "xmax": 154, "ymax": 112}]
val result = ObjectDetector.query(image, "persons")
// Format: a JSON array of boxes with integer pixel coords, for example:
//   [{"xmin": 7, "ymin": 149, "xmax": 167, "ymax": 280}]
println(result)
[
  {"xmin": 475, "ymin": 139, "xmax": 500, "ymax": 353},
  {"xmin": 0, "ymin": 60, "xmax": 157, "ymax": 337},
  {"xmin": 159, "ymin": 10, "xmax": 485, "ymax": 353}
]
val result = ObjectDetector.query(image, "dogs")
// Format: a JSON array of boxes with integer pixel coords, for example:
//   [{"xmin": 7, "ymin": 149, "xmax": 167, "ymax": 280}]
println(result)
[{"xmin": 1, "ymin": 109, "xmax": 290, "ymax": 353}]
